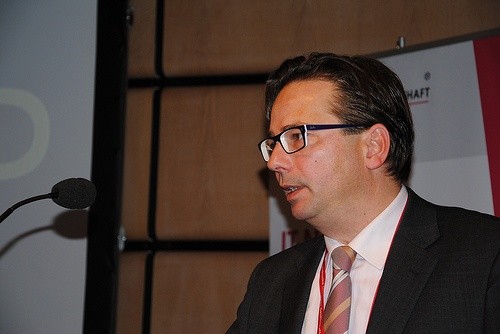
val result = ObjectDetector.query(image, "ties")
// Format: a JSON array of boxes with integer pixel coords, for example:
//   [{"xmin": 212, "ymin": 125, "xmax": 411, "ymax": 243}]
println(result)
[{"xmin": 319, "ymin": 246, "xmax": 358, "ymax": 334}]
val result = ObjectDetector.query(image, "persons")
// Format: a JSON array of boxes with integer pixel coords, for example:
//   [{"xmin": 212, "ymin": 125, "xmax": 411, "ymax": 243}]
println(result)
[{"xmin": 223, "ymin": 51, "xmax": 500, "ymax": 334}]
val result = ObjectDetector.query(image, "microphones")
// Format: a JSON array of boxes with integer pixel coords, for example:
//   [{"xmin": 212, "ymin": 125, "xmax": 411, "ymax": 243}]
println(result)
[{"xmin": 0, "ymin": 177, "xmax": 96, "ymax": 223}]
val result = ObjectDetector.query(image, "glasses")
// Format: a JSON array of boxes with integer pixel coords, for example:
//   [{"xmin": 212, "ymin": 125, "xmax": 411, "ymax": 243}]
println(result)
[{"xmin": 257, "ymin": 120, "xmax": 378, "ymax": 162}]
